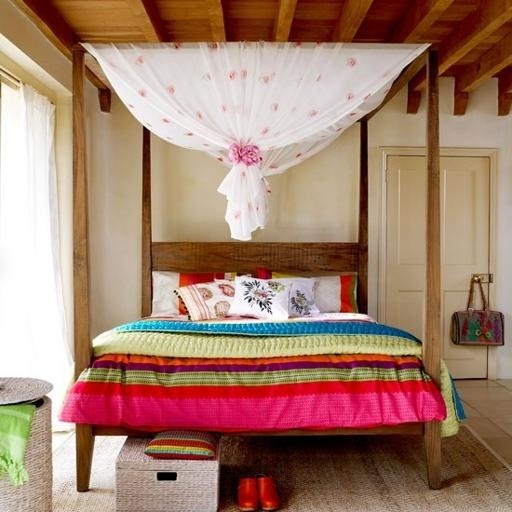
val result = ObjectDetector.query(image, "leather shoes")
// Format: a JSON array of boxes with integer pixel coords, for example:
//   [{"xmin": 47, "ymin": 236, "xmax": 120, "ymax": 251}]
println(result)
[
  {"xmin": 237, "ymin": 474, "xmax": 258, "ymax": 511},
  {"xmin": 256, "ymin": 474, "xmax": 281, "ymax": 510}
]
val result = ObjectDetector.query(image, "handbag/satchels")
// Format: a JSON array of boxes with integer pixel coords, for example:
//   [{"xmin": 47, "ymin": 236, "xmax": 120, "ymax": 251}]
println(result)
[{"xmin": 452, "ymin": 274, "xmax": 507, "ymax": 347}]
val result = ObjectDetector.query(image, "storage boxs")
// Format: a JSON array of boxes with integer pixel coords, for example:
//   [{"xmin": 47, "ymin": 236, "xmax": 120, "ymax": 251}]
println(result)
[{"xmin": 115, "ymin": 436, "xmax": 221, "ymax": 512}]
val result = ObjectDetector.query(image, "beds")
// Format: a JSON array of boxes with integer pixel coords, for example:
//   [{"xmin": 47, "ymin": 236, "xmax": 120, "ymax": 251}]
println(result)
[{"xmin": 67, "ymin": 36, "xmax": 455, "ymax": 492}]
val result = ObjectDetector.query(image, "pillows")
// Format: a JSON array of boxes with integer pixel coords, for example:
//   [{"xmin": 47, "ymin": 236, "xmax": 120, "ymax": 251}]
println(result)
[
  {"xmin": 258, "ymin": 268, "xmax": 363, "ymax": 312},
  {"xmin": 276, "ymin": 279, "xmax": 321, "ymax": 318},
  {"xmin": 148, "ymin": 271, "xmax": 253, "ymax": 318},
  {"xmin": 230, "ymin": 277, "xmax": 293, "ymax": 318},
  {"xmin": 171, "ymin": 283, "xmax": 236, "ymax": 319},
  {"xmin": 144, "ymin": 428, "xmax": 215, "ymax": 459}
]
